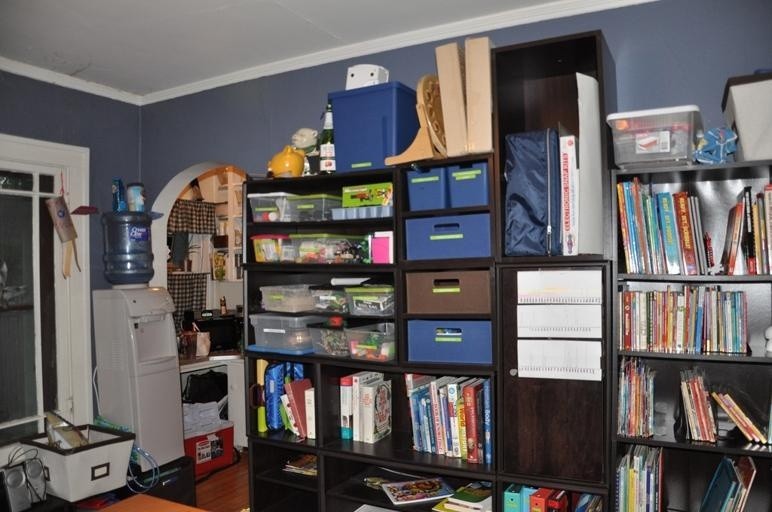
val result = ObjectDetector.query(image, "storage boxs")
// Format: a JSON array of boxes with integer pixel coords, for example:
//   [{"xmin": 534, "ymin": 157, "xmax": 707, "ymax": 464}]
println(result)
[
  {"xmin": 260, "ymin": 284, "xmax": 317, "ymax": 313},
  {"xmin": 406, "ymin": 270, "xmax": 491, "ymax": 314},
  {"xmin": 447, "ymin": 163, "xmax": 489, "ymax": 209},
  {"xmin": 406, "ymin": 214, "xmax": 492, "ymax": 261},
  {"xmin": 343, "ymin": 285, "xmax": 395, "ymax": 317},
  {"xmin": 372, "ymin": 231, "xmax": 393, "ymax": 264},
  {"xmin": 289, "ymin": 234, "xmax": 372, "ymax": 263},
  {"xmin": 407, "ymin": 168, "xmax": 449, "ymax": 212},
  {"xmin": 327, "ymin": 83, "xmax": 419, "ymax": 170},
  {"xmin": 286, "ymin": 194, "xmax": 343, "ymax": 221},
  {"xmin": 306, "ymin": 320, "xmax": 376, "ymax": 357},
  {"xmin": 19, "ymin": 423, "xmax": 136, "ymax": 502},
  {"xmin": 342, "ymin": 181, "xmax": 394, "ymax": 207},
  {"xmin": 720, "ymin": 72, "xmax": 772, "ymax": 162},
  {"xmin": 309, "ymin": 283, "xmax": 364, "ymax": 314},
  {"xmin": 603, "ymin": 106, "xmax": 703, "ymax": 165},
  {"xmin": 251, "ymin": 234, "xmax": 295, "ymax": 263},
  {"xmin": 407, "ymin": 321, "xmax": 493, "ymax": 364},
  {"xmin": 344, "ymin": 323, "xmax": 394, "ymax": 362},
  {"xmin": 247, "ymin": 192, "xmax": 300, "ymax": 222},
  {"xmin": 185, "ymin": 401, "xmax": 233, "ymax": 482},
  {"xmin": 249, "ymin": 314, "xmax": 328, "ymax": 356}
]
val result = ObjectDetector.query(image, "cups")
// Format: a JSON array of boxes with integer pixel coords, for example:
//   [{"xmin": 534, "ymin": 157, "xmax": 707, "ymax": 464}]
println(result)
[
  {"xmin": 236, "ymin": 305, "xmax": 243, "ymax": 312},
  {"xmin": 182, "ymin": 331, "xmax": 209, "ymax": 362},
  {"xmin": 126, "ymin": 182, "xmax": 146, "ymax": 213}
]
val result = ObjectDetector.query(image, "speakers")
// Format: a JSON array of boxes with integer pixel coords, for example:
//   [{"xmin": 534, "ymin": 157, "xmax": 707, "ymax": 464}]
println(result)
[{"xmin": 0, "ymin": 458, "xmax": 47, "ymax": 512}]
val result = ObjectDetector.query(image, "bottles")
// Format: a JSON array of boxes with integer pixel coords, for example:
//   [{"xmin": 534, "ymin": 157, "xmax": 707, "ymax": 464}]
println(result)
[
  {"xmin": 316, "ymin": 103, "xmax": 335, "ymax": 175},
  {"xmin": 110, "ymin": 176, "xmax": 126, "ymax": 209}
]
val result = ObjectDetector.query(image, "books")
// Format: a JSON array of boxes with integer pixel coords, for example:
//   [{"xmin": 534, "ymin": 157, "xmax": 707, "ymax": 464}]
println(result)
[
  {"xmin": 355, "ymin": 477, "xmax": 492, "ymax": 511},
  {"xmin": 283, "ymin": 452, "xmax": 318, "ymax": 475},
  {"xmin": 700, "ymin": 456, "xmax": 756, "ymax": 511},
  {"xmin": 618, "ymin": 175, "xmax": 772, "ymax": 276},
  {"xmin": 680, "ymin": 366, "xmax": 772, "ymax": 445},
  {"xmin": 279, "ymin": 378, "xmax": 315, "ymax": 439},
  {"xmin": 616, "ymin": 443, "xmax": 663, "ymax": 512},
  {"xmin": 618, "ymin": 284, "xmax": 748, "ymax": 354},
  {"xmin": 617, "ymin": 357, "xmax": 654, "ymax": 437},
  {"xmin": 504, "ymin": 484, "xmax": 602, "ymax": 511},
  {"xmin": 341, "ymin": 370, "xmax": 392, "ymax": 444},
  {"xmin": 405, "ymin": 373, "xmax": 495, "ymax": 464}
]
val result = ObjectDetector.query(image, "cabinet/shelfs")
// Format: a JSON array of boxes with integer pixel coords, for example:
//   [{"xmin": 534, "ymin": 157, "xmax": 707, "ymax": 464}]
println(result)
[
  {"xmin": 208, "ymin": 172, "xmax": 244, "ymax": 283},
  {"xmin": 242, "ymin": 29, "xmax": 616, "ymax": 512},
  {"xmin": 610, "ymin": 159, "xmax": 770, "ymax": 507}
]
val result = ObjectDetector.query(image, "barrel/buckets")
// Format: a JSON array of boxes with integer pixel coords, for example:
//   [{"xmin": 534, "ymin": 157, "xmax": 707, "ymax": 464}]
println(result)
[{"xmin": 102, "ymin": 211, "xmax": 154, "ymax": 283}]
